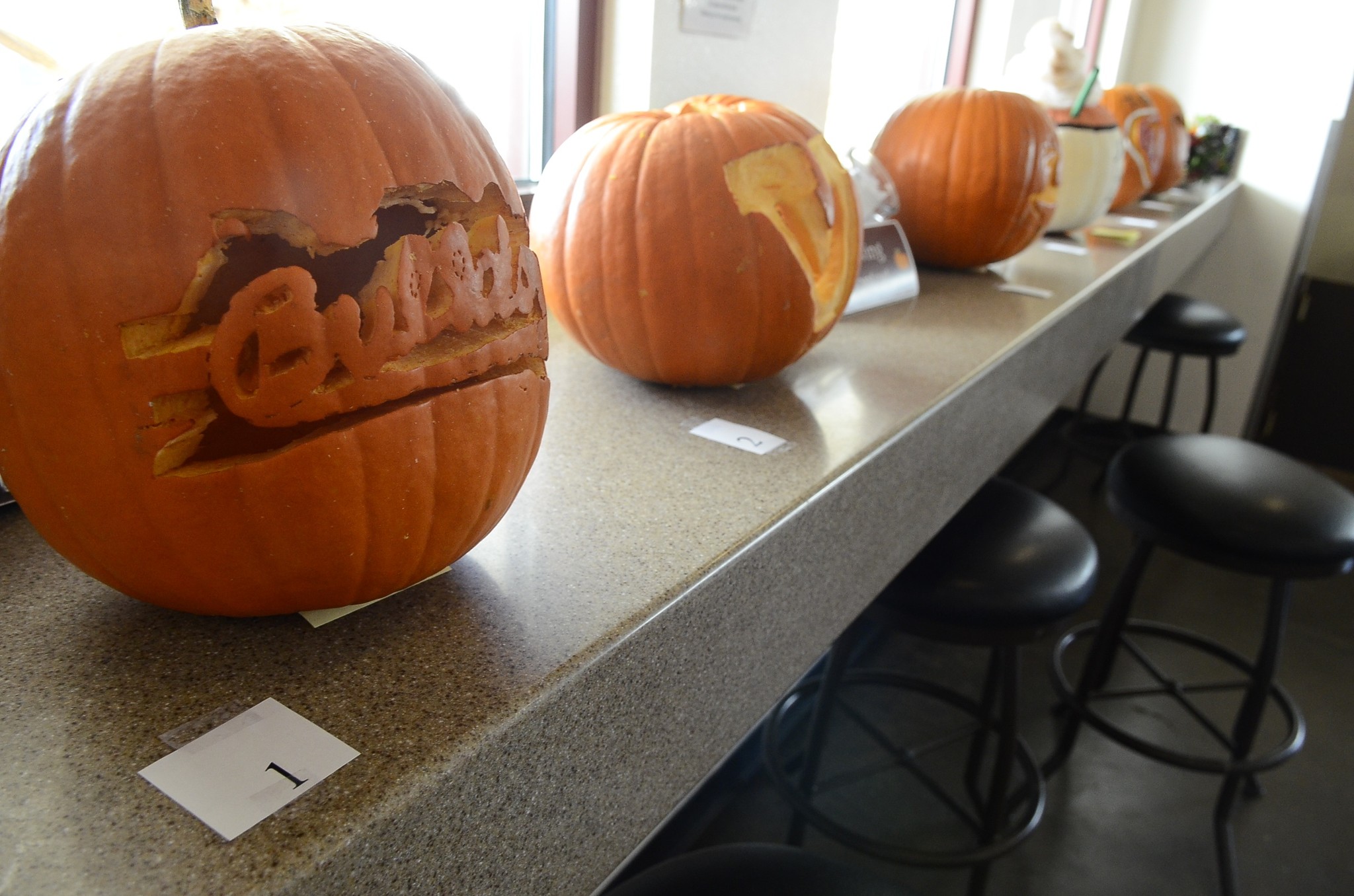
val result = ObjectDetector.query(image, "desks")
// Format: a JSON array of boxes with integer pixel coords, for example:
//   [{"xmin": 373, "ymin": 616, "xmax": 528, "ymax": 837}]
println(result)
[{"xmin": 1, "ymin": 173, "xmax": 1242, "ymax": 896}]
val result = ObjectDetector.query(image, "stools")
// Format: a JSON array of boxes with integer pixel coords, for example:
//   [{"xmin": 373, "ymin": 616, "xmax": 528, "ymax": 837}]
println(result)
[
  {"xmin": 737, "ymin": 478, "xmax": 1100, "ymax": 896},
  {"xmin": 1016, "ymin": 431, "xmax": 1353, "ymax": 896},
  {"xmin": 600, "ymin": 841, "xmax": 919, "ymax": 895},
  {"xmin": 1043, "ymin": 293, "xmax": 1247, "ymax": 508}
]
